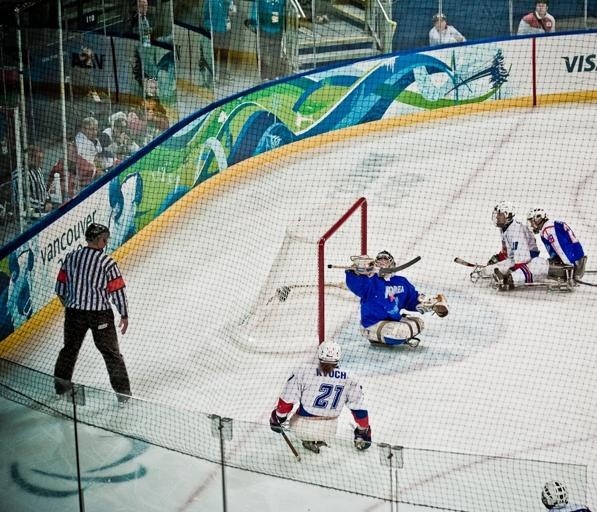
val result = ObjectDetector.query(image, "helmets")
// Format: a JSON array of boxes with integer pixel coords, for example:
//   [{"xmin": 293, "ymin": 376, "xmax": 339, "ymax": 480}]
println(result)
[
  {"xmin": 432, "ymin": 14, "xmax": 447, "ymax": 22},
  {"xmin": 315, "ymin": 339, "xmax": 343, "ymax": 364},
  {"xmin": 84, "ymin": 222, "xmax": 110, "ymax": 241},
  {"xmin": 373, "ymin": 249, "xmax": 396, "ymax": 268},
  {"xmin": 494, "ymin": 200, "xmax": 515, "ymax": 219},
  {"xmin": 540, "ymin": 479, "xmax": 569, "ymax": 506},
  {"xmin": 525, "ymin": 207, "xmax": 547, "ymax": 221}
]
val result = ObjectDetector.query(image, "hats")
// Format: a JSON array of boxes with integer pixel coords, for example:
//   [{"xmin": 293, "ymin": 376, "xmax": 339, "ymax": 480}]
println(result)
[{"xmin": 113, "ymin": 117, "xmax": 129, "ymax": 132}]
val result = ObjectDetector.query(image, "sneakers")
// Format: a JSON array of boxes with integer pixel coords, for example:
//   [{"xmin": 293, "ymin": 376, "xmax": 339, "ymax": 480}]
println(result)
[
  {"xmin": 116, "ymin": 391, "xmax": 132, "ymax": 403},
  {"xmin": 54, "ymin": 381, "xmax": 75, "ymax": 394},
  {"xmin": 493, "ymin": 268, "xmax": 515, "ymax": 291}
]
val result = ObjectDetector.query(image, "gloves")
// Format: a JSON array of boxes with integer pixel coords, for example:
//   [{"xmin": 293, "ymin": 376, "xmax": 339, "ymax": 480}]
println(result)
[
  {"xmin": 349, "ymin": 253, "xmax": 375, "ymax": 278},
  {"xmin": 352, "ymin": 425, "xmax": 372, "ymax": 450},
  {"xmin": 486, "ymin": 254, "xmax": 499, "ymax": 266},
  {"xmin": 268, "ymin": 409, "xmax": 287, "ymax": 433},
  {"xmin": 562, "ymin": 266, "xmax": 581, "ymax": 289},
  {"xmin": 416, "ymin": 292, "xmax": 449, "ymax": 319}
]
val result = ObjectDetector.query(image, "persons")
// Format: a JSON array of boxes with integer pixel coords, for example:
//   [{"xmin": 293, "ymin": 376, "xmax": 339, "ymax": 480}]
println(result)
[
  {"xmin": 0, "ymin": 81, "xmax": 168, "ymax": 223},
  {"xmin": 52, "ymin": 223, "xmax": 130, "ymax": 402},
  {"xmin": 270, "ymin": 340, "xmax": 369, "ymax": 450},
  {"xmin": 540, "ymin": 479, "xmax": 590, "ymax": 512},
  {"xmin": 197, "ymin": 0, "xmax": 235, "ymax": 91},
  {"xmin": 311, "ymin": 0, "xmax": 335, "ymax": 28},
  {"xmin": 494, "ymin": 206, "xmax": 587, "ymax": 292},
  {"xmin": 124, "ymin": 0, "xmax": 155, "ymax": 46},
  {"xmin": 4, "ymin": 241, "xmax": 35, "ymax": 333},
  {"xmin": 248, "ymin": 1, "xmax": 288, "ymax": 83},
  {"xmin": 105, "ymin": 174, "xmax": 137, "ymax": 257},
  {"xmin": 515, "ymin": 0, "xmax": 556, "ymax": 37},
  {"xmin": 428, "ymin": 14, "xmax": 465, "ymax": 47},
  {"xmin": 476, "ymin": 201, "xmax": 540, "ymax": 281},
  {"xmin": 344, "ymin": 250, "xmax": 449, "ymax": 350}
]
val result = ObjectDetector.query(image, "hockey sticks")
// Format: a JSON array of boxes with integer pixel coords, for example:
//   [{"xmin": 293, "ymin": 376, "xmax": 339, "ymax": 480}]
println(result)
[
  {"xmin": 454, "ymin": 257, "xmax": 488, "ymax": 268},
  {"xmin": 328, "ymin": 256, "xmax": 421, "ymax": 273},
  {"xmin": 279, "ymin": 422, "xmax": 312, "ymax": 467}
]
